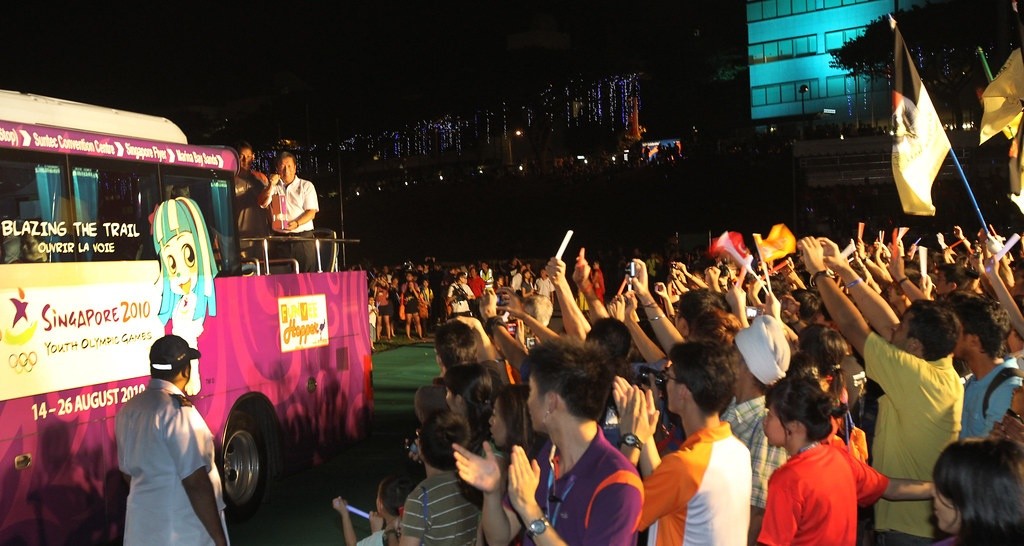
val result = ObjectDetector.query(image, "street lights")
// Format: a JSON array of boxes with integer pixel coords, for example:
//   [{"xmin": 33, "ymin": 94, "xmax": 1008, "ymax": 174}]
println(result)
[{"xmin": 800, "ymin": 85, "xmax": 809, "ymax": 140}]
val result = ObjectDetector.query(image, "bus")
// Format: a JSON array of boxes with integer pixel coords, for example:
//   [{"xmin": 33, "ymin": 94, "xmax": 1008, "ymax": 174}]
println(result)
[{"xmin": 0, "ymin": 87, "xmax": 377, "ymax": 546}]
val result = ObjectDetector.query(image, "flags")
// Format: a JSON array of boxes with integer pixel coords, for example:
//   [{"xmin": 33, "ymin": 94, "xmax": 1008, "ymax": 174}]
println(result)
[
  {"xmin": 753, "ymin": 224, "xmax": 796, "ymax": 267},
  {"xmin": 977, "ymin": 48, "xmax": 1024, "ymax": 146},
  {"xmin": 890, "ymin": 18, "xmax": 953, "ymax": 217},
  {"xmin": 708, "ymin": 231, "xmax": 754, "ymax": 267}
]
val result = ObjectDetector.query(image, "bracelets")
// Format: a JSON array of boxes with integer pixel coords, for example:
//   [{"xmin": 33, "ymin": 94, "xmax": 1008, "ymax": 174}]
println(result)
[
  {"xmin": 845, "ymin": 277, "xmax": 864, "ymax": 288},
  {"xmin": 861, "ymin": 257, "xmax": 869, "ymax": 261},
  {"xmin": 384, "ymin": 529, "xmax": 398, "ymax": 541},
  {"xmin": 294, "ymin": 220, "xmax": 299, "ymax": 227},
  {"xmin": 486, "ymin": 316, "xmax": 506, "ymax": 338},
  {"xmin": 647, "ymin": 313, "xmax": 666, "ymax": 321},
  {"xmin": 898, "ymin": 277, "xmax": 909, "ymax": 284}
]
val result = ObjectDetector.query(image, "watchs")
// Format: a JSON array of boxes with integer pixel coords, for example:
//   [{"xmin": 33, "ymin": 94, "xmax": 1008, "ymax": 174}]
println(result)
[
  {"xmin": 620, "ymin": 433, "xmax": 644, "ymax": 451},
  {"xmin": 525, "ymin": 515, "xmax": 549, "ymax": 538},
  {"xmin": 810, "ymin": 267, "xmax": 835, "ymax": 288}
]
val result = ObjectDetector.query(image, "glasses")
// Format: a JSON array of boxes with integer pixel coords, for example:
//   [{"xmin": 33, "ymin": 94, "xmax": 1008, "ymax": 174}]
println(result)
[{"xmin": 662, "ymin": 372, "xmax": 678, "ymax": 381}]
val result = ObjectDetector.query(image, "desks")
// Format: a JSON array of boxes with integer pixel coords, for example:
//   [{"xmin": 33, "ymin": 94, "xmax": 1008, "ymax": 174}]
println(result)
[{"xmin": 242, "ymin": 236, "xmax": 360, "ymax": 274}]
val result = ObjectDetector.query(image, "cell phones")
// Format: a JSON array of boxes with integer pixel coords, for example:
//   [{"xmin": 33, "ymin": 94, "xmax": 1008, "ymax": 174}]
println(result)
[
  {"xmin": 526, "ymin": 338, "xmax": 536, "ymax": 349},
  {"xmin": 507, "ymin": 323, "xmax": 517, "ymax": 334}
]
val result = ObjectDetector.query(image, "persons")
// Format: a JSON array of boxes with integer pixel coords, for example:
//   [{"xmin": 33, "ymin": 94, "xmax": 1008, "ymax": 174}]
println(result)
[{"xmin": 0, "ymin": 123, "xmax": 1024, "ymax": 546}]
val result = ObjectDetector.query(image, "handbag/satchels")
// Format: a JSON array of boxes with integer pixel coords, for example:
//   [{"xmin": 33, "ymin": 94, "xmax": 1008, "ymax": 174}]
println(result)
[{"xmin": 848, "ymin": 425, "xmax": 869, "ymax": 463}]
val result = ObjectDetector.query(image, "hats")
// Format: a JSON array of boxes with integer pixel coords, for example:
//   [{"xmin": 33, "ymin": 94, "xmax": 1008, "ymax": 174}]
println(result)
[
  {"xmin": 150, "ymin": 335, "xmax": 200, "ymax": 370},
  {"xmin": 735, "ymin": 315, "xmax": 790, "ymax": 385}
]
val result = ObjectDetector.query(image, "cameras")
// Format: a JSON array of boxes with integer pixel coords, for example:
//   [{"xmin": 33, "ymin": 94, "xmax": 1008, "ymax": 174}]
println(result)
[
  {"xmin": 745, "ymin": 306, "xmax": 763, "ymax": 319},
  {"xmin": 495, "ymin": 293, "xmax": 503, "ymax": 306},
  {"xmin": 637, "ymin": 366, "xmax": 667, "ymax": 390},
  {"xmin": 625, "ymin": 262, "xmax": 635, "ymax": 279}
]
